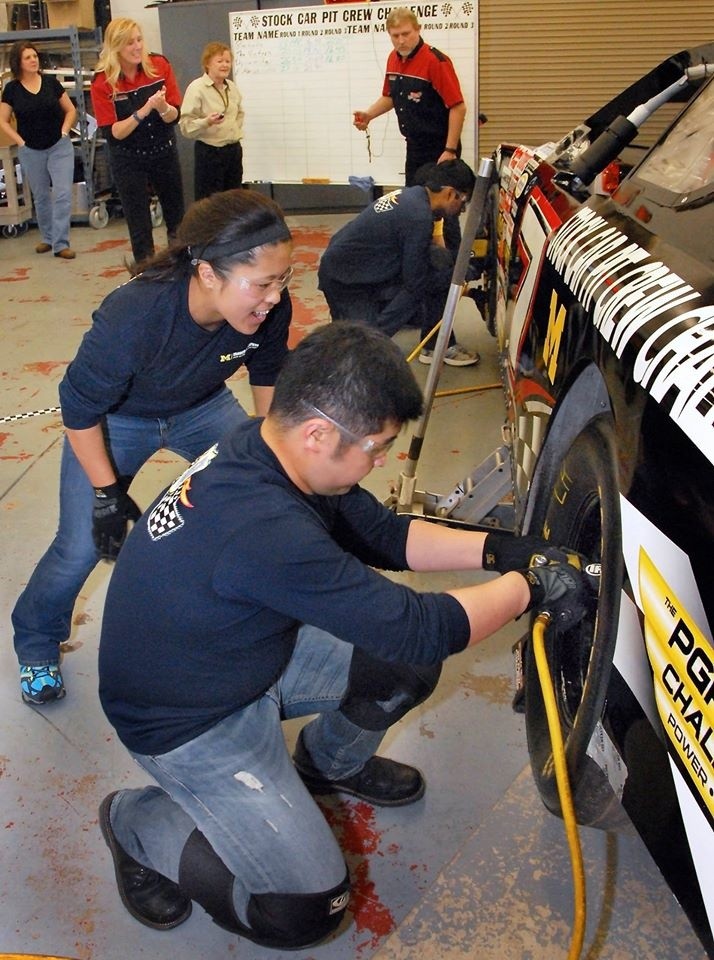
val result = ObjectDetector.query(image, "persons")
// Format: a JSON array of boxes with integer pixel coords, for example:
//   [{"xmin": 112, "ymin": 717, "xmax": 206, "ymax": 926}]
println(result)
[
  {"xmin": 98, "ymin": 319, "xmax": 602, "ymax": 951},
  {"xmin": 177, "ymin": 42, "xmax": 245, "ymax": 201},
  {"xmin": 0, "ymin": 41, "xmax": 78, "ymax": 258},
  {"xmin": 316, "ymin": 158, "xmax": 481, "ymax": 367},
  {"xmin": 10, "ymin": 192, "xmax": 293, "ymax": 707},
  {"xmin": 351, "ymin": 9, "xmax": 466, "ymax": 265},
  {"xmin": 90, "ymin": 18, "xmax": 183, "ymax": 266}
]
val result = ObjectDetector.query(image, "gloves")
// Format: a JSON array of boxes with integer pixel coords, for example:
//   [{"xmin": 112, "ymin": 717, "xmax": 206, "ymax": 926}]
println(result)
[
  {"xmin": 481, "ymin": 532, "xmax": 590, "ymax": 576},
  {"xmin": 506, "ymin": 561, "xmax": 590, "ymax": 633},
  {"xmin": 93, "ymin": 479, "xmax": 144, "ymax": 559}
]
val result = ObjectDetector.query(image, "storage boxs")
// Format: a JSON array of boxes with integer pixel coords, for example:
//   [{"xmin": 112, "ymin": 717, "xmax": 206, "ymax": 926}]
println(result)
[{"xmin": 70, "ymin": 181, "xmax": 90, "ymax": 213}]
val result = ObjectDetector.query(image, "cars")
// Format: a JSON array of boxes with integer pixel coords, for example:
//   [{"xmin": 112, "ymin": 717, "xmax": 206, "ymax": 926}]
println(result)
[{"xmin": 494, "ymin": 45, "xmax": 714, "ymax": 948}]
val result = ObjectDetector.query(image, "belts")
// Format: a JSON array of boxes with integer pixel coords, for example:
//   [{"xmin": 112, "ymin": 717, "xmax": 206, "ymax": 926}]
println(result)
[{"xmin": 115, "ymin": 139, "xmax": 175, "ymax": 155}]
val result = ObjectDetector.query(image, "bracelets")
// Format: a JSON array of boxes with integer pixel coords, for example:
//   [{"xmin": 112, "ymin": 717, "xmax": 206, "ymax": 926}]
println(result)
[
  {"xmin": 62, "ymin": 132, "xmax": 67, "ymax": 136},
  {"xmin": 134, "ymin": 111, "xmax": 142, "ymax": 123},
  {"xmin": 444, "ymin": 147, "xmax": 457, "ymax": 154},
  {"xmin": 159, "ymin": 105, "xmax": 171, "ymax": 116}
]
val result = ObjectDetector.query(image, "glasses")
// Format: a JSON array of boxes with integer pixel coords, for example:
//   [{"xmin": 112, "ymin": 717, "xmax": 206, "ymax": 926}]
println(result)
[
  {"xmin": 297, "ymin": 397, "xmax": 401, "ymax": 462},
  {"xmin": 212, "ymin": 263, "xmax": 294, "ymax": 296},
  {"xmin": 441, "ymin": 185, "xmax": 471, "ymax": 209}
]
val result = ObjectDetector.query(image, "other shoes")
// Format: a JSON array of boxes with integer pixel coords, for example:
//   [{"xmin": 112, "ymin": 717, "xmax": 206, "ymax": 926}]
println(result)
[
  {"xmin": 55, "ymin": 247, "xmax": 76, "ymax": 259},
  {"xmin": 36, "ymin": 243, "xmax": 52, "ymax": 253}
]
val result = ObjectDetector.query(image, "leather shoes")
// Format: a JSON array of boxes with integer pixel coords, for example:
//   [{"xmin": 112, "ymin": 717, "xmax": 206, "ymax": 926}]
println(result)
[
  {"xmin": 291, "ymin": 753, "xmax": 425, "ymax": 807},
  {"xmin": 98, "ymin": 789, "xmax": 193, "ymax": 931}
]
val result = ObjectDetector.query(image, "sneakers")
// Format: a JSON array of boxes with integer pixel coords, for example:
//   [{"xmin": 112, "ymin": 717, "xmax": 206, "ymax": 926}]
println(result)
[
  {"xmin": 418, "ymin": 344, "xmax": 480, "ymax": 366},
  {"xmin": 18, "ymin": 663, "xmax": 66, "ymax": 705}
]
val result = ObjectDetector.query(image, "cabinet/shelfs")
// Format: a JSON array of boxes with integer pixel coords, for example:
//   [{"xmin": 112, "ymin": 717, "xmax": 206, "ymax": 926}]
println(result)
[{"xmin": 0, "ymin": 25, "xmax": 113, "ymax": 204}]
